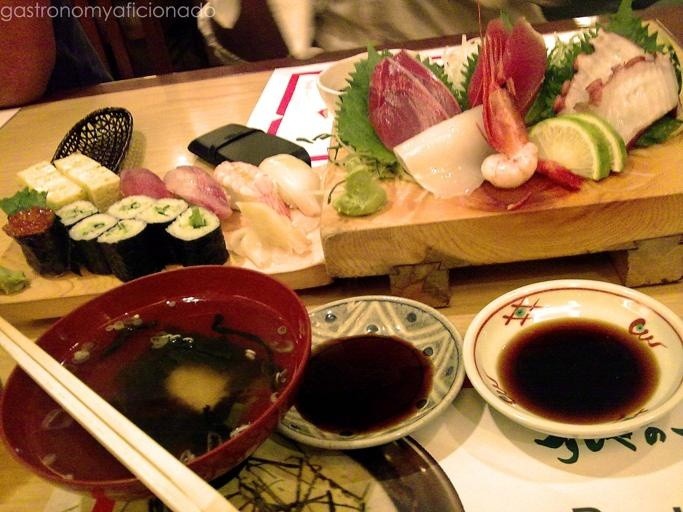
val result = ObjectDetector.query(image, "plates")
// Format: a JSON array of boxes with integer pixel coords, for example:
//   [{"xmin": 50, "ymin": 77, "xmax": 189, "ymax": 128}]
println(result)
[
  {"xmin": 461, "ymin": 279, "xmax": 683, "ymax": 442},
  {"xmin": 280, "ymin": 293, "xmax": 467, "ymax": 452}
]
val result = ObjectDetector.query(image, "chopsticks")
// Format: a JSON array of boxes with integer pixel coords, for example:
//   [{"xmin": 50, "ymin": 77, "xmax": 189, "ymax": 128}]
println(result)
[{"xmin": 0, "ymin": 316, "xmax": 243, "ymax": 512}]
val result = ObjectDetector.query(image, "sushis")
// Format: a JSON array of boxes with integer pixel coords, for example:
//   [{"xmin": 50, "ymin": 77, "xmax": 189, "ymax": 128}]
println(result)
[{"xmin": 2, "ymin": 196, "xmax": 227, "ymax": 281}]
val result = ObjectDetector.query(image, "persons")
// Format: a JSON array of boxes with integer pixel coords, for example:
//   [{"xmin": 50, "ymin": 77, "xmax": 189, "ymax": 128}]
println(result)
[{"xmin": 0, "ymin": 0, "xmax": 212, "ymax": 108}]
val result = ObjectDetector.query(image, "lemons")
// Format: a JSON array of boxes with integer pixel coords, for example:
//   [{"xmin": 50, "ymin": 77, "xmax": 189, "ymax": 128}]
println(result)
[{"xmin": 529, "ymin": 114, "xmax": 625, "ymax": 180}]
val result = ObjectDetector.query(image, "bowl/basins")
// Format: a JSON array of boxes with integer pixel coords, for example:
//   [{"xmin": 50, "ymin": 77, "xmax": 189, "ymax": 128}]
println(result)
[
  {"xmin": 312, "ymin": 46, "xmax": 416, "ymax": 118},
  {"xmin": 0, "ymin": 263, "xmax": 314, "ymax": 502}
]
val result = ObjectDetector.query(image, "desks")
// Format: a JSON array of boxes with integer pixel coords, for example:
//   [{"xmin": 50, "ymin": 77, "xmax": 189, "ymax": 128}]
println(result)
[{"xmin": 0, "ymin": 4, "xmax": 683, "ymax": 512}]
